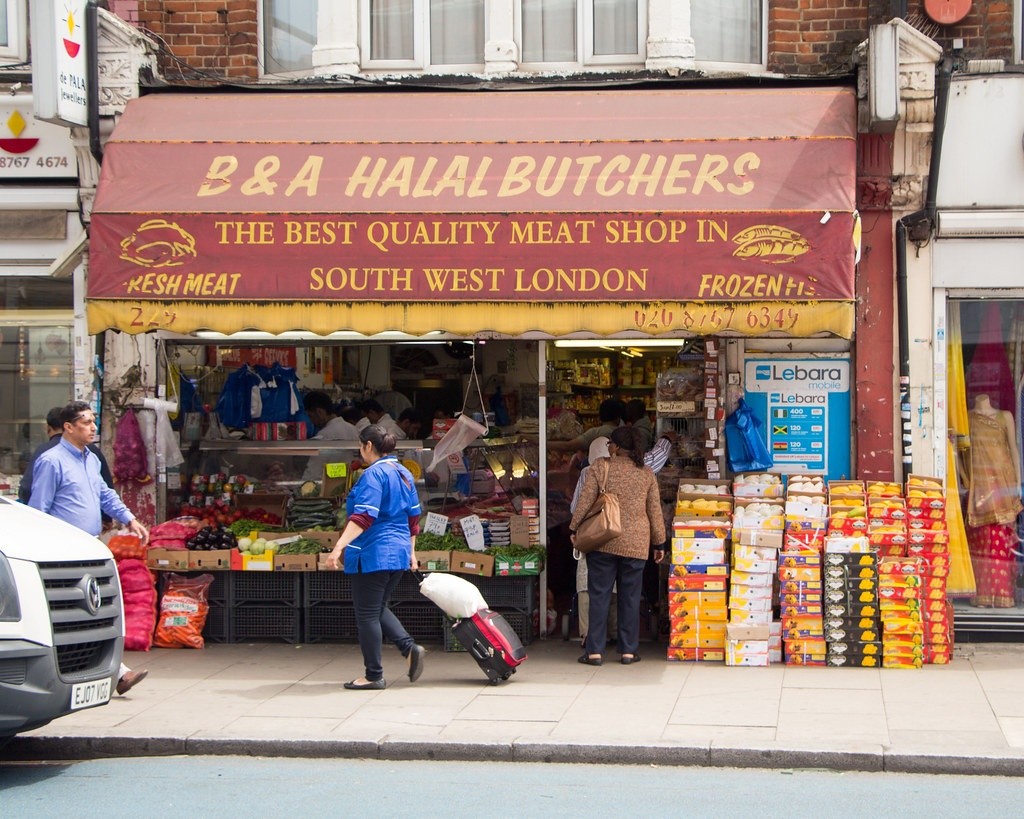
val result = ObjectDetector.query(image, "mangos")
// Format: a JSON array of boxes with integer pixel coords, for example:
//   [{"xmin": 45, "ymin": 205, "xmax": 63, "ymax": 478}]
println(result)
[{"xmin": 847, "ymin": 506, "xmax": 866, "ymax": 518}]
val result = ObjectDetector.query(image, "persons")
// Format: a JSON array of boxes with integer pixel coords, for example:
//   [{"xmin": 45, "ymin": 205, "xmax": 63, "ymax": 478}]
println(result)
[
  {"xmin": 570, "ymin": 431, "xmax": 677, "ymax": 648},
  {"xmin": 431, "ymin": 404, "xmax": 449, "ymax": 419},
  {"xmin": 342, "ymin": 399, "xmax": 424, "ymax": 438},
  {"xmin": 570, "ymin": 426, "xmax": 667, "ymax": 666},
  {"xmin": 240, "ymin": 454, "xmax": 289, "ymax": 481},
  {"xmin": 27, "ymin": 400, "xmax": 149, "ymax": 695},
  {"xmin": 325, "ymin": 425, "xmax": 425, "ymax": 690},
  {"xmin": 578, "ymin": 396, "xmax": 653, "ymax": 441},
  {"xmin": 957, "ymin": 394, "xmax": 1024, "ymax": 608},
  {"xmin": 18, "ymin": 406, "xmax": 113, "ymax": 535},
  {"xmin": 303, "ymin": 391, "xmax": 361, "ymax": 481}
]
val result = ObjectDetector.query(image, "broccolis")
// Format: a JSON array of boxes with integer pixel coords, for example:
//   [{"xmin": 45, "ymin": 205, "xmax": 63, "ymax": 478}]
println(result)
[{"xmin": 301, "ymin": 482, "xmax": 316, "ymax": 495}]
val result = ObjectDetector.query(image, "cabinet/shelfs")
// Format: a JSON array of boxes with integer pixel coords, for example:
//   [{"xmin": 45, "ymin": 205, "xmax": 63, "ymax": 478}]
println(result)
[
  {"xmin": 656, "ymin": 373, "xmax": 706, "ymax": 646},
  {"xmin": 618, "ymin": 384, "xmax": 655, "ymax": 410},
  {"xmin": 546, "ymin": 381, "xmax": 615, "ymax": 416}
]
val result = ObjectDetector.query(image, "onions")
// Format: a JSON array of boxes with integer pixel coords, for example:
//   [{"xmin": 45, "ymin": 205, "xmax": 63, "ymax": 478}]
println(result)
[
  {"xmin": 235, "ymin": 536, "xmax": 278, "ymax": 557},
  {"xmin": 112, "ymin": 438, "xmax": 148, "ymax": 480}
]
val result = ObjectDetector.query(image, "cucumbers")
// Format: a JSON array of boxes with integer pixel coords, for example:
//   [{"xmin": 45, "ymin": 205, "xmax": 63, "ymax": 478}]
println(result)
[{"xmin": 286, "ymin": 498, "xmax": 336, "ymax": 530}]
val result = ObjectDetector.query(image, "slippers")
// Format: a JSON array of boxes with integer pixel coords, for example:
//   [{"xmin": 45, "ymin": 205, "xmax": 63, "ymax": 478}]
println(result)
[
  {"xmin": 578, "ymin": 653, "xmax": 602, "ymax": 666},
  {"xmin": 621, "ymin": 653, "xmax": 641, "ymax": 664}
]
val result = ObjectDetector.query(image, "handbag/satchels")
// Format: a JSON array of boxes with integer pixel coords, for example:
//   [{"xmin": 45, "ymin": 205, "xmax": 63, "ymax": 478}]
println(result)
[
  {"xmin": 418, "ymin": 573, "xmax": 488, "ymax": 618},
  {"xmin": 573, "ymin": 460, "xmax": 621, "ymax": 554}
]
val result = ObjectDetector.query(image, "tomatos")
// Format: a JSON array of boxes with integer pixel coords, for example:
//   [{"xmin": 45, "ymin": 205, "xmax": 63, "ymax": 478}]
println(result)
[{"xmin": 182, "ymin": 473, "xmax": 246, "ymax": 527}]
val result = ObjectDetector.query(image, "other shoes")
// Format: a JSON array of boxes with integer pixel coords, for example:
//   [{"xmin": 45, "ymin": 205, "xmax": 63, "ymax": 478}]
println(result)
[
  {"xmin": 409, "ymin": 645, "xmax": 425, "ymax": 682},
  {"xmin": 343, "ymin": 678, "xmax": 386, "ymax": 689}
]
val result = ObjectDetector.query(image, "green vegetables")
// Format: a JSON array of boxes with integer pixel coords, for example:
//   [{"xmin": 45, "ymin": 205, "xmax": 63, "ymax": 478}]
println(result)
[{"xmin": 414, "ymin": 530, "xmax": 545, "ymax": 556}]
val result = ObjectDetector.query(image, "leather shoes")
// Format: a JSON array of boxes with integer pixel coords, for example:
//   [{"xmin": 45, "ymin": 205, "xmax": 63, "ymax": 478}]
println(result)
[{"xmin": 116, "ymin": 669, "xmax": 149, "ymax": 695}]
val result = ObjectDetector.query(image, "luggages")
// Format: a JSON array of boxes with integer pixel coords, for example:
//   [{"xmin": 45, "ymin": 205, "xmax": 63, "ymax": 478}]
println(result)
[{"xmin": 409, "ymin": 567, "xmax": 528, "ymax": 685}]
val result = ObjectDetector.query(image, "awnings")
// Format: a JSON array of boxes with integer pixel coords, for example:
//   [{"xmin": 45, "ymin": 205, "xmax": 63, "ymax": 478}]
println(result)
[{"xmin": 85, "ymin": 83, "xmax": 857, "ymax": 339}]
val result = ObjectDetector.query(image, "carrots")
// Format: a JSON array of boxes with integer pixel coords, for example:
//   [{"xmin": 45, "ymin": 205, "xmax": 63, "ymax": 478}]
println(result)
[{"xmin": 154, "ymin": 591, "xmax": 208, "ymax": 648}]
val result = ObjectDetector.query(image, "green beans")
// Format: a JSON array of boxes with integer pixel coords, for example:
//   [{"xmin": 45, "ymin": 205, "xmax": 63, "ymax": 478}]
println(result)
[{"xmin": 279, "ymin": 537, "xmax": 323, "ymax": 554}]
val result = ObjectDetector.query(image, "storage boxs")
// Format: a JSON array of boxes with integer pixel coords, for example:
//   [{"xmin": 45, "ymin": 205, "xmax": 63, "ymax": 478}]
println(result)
[
  {"xmin": 433, "ymin": 418, "xmax": 458, "ymax": 438},
  {"xmin": 666, "ymin": 472, "xmax": 954, "ymax": 669},
  {"xmin": 145, "ymin": 462, "xmax": 542, "ymax": 651},
  {"xmin": 247, "ymin": 421, "xmax": 307, "ymax": 440}
]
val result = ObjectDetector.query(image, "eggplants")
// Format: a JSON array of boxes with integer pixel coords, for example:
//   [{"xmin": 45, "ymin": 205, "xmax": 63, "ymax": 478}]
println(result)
[{"xmin": 185, "ymin": 522, "xmax": 238, "ymax": 550}]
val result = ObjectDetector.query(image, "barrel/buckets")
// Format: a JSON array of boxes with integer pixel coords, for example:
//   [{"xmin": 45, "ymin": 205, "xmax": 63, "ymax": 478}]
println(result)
[{"xmin": 472, "ymin": 412, "xmax": 495, "ymax": 426}]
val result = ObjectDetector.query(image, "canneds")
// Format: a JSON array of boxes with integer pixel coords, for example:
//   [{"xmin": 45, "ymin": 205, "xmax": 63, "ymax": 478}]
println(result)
[{"xmin": 577, "ymin": 355, "xmax": 674, "ymax": 386}]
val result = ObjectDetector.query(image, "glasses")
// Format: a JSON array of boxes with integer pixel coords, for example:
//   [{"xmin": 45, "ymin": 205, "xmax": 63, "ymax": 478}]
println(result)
[
  {"xmin": 606, "ymin": 441, "xmax": 613, "ymax": 447},
  {"xmin": 69, "ymin": 413, "xmax": 96, "ymax": 422}
]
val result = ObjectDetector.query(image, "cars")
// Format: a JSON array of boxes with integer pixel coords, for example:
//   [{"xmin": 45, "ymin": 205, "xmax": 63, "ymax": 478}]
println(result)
[{"xmin": 0, "ymin": 492, "xmax": 127, "ymax": 739}]
